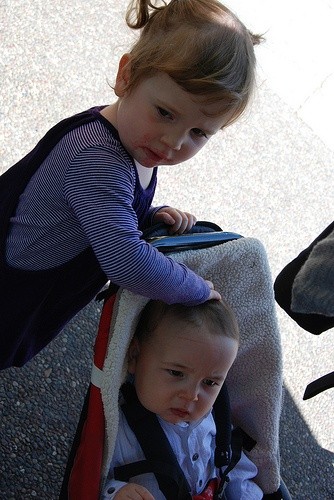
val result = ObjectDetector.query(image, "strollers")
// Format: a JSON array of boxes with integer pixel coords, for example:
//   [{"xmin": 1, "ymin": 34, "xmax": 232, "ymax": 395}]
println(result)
[{"xmin": 59, "ymin": 219, "xmax": 292, "ymax": 499}]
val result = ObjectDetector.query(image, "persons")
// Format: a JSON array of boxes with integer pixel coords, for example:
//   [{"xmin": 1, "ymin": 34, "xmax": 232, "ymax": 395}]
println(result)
[
  {"xmin": 104, "ymin": 299, "xmax": 266, "ymax": 500},
  {"xmin": 0, "ymin": 0, "xmax": 272, "ymax": 378}
]
